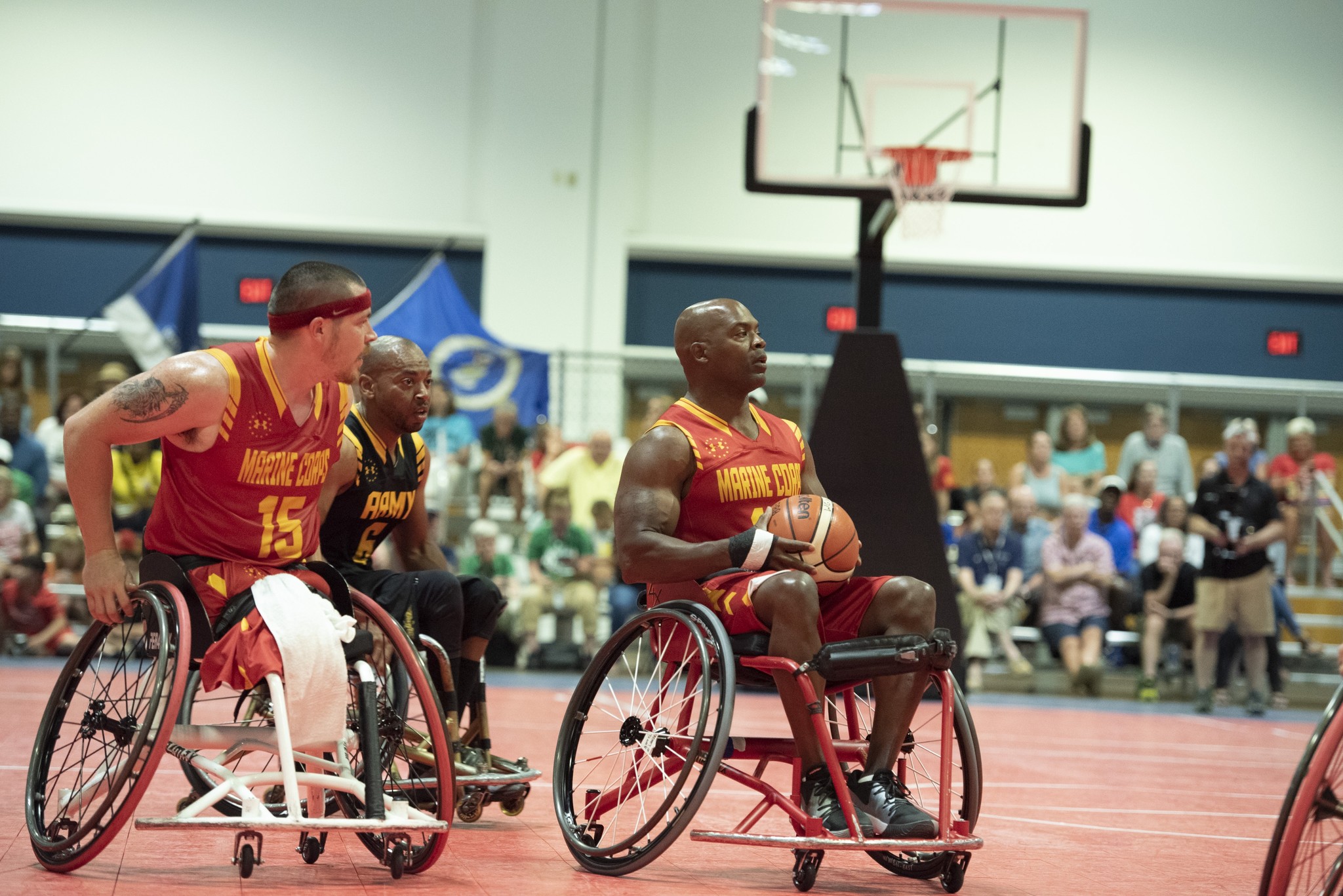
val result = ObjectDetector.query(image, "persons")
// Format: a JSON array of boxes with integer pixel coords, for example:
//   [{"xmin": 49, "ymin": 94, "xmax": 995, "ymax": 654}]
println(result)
[
  {"xmin": 1184, "ymin": 418, "xmax": 1290, "ymax": 717},
  {"xmin": 612, "ymin": 298, "xmax": 938, "ymax": 838},
  {"xmin": 62, "ymin": 261, "xmax": 397, "ymax": 675},
  {"xmin": 0, "ymin": 325, "xmax": 1339, "ymax": 703}
]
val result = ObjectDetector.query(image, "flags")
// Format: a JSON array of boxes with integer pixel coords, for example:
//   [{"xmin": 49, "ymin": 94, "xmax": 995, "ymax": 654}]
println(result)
[
  {"xmin": 363, "ymin": 253, "xmax": 549, "ymax": 441},
  {"xmin": 103, "ymin": 222, "xmax": 203, "ymax": 372}
]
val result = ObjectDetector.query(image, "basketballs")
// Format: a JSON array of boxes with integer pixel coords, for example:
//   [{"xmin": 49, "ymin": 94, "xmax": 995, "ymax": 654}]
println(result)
[{"xmin": 767, "ymin": 494, "xmax": 859, "ymax": 597}]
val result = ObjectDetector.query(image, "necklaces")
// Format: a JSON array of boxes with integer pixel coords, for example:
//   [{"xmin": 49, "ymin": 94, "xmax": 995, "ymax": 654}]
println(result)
[{"xmin": 301, "ymin": 334, "xmax": 532, "ymax": 804}]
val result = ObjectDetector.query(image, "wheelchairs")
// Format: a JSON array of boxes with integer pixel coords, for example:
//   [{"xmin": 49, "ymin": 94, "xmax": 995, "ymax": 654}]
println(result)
[
  {"xmin": 23, "ymin": 548, "xmax": 544, "ymax": 879},
  {"xmin": 1260, "ymin": 678, "xmax": 1343, "ymax": 896},
  {"xmin": 550, "ymin": 582, "xmax": 987, "ymax": 896}
]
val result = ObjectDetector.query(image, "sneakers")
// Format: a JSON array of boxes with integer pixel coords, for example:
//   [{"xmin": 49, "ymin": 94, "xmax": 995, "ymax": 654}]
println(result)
[
  {"xmin": 800, "ymin": 770, "xmax": 873, "ymax": 839},
  {"xmin": 848, "ymin": 768, "xmax": 939, "ymax": 839}
]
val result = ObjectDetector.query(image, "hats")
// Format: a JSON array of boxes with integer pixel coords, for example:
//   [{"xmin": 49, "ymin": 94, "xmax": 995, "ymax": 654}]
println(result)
[
  {"xmin": 1100, "ymin": 475, "xmax": 1129, "ymax": 494},
  {"xmin": 96, "ymin": 361, "xmax": 130, "ymax": 383}
]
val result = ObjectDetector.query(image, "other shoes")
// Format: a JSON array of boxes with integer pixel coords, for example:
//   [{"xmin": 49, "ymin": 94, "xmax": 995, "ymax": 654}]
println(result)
[
  {"xmin": 408, "ymin": 766, "xmax": 437, "ymax": 804},
  {"xmin": 464, "ymin": 782, "xmax": 526, "ymax": 801},
  {"xmin": 1137, "ymin": 679, "xmax": 1158, "ymax": 698},
  {"xmin": 1194, "ymin": 691, "xmax": 1210, "ymax": 712},
  {"xmin": 1249, "ymin": 693, "xmax": 1265, "ymax": 714},
  {"xmin": 1073, "ymin": 663, "xmax": 1103, "ymax": 696}
]
